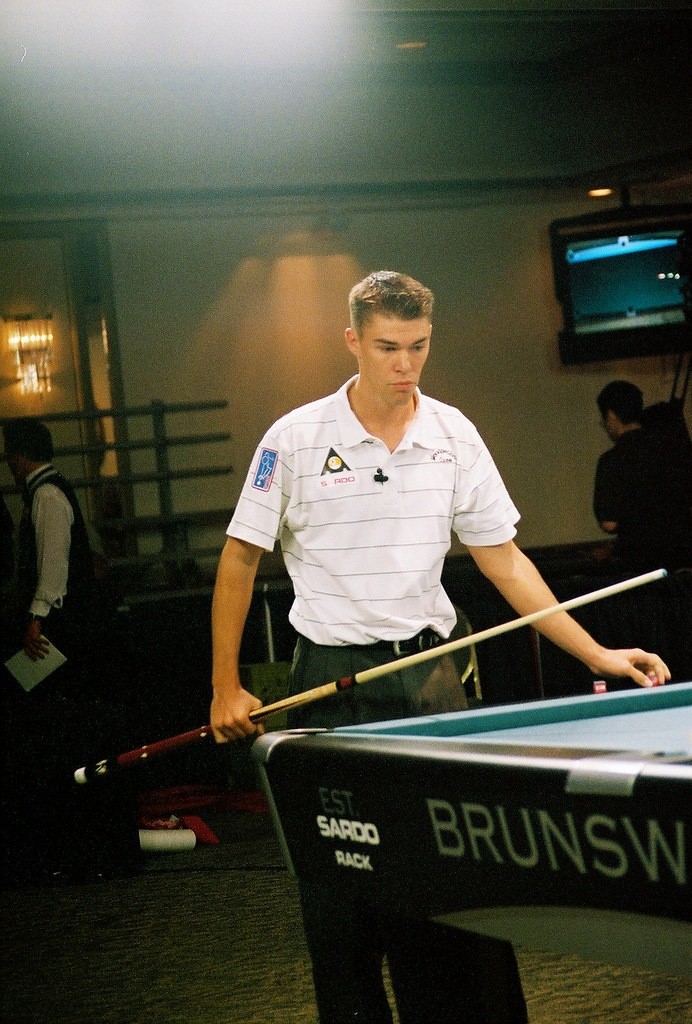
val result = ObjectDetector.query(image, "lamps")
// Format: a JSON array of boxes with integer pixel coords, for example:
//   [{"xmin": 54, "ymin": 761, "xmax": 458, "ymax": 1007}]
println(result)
[{"xmin": 5, "ymin": 313, "xmax": 53, "ymax": 395}]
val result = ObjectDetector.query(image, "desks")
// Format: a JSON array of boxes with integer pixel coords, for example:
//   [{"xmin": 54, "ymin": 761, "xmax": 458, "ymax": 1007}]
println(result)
[
  {"xmin": 250, "ymin": 682, "xmax": 692, "ymax": 1024},
  {"xmin": 119, "ymin": 536, "xmax": 624, "ymax": 700}
]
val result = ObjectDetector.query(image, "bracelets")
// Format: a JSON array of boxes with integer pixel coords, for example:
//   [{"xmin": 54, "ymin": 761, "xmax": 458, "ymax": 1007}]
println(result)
[{"xmin": 26, "ymin": 612, "xmax": 45, "ymax": 621}]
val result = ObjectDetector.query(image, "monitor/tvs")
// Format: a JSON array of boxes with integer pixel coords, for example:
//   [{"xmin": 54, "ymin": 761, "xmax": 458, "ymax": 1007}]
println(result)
[{"xmin": 553, "ymin": 219, "xmax": 692, "ymax": 367}]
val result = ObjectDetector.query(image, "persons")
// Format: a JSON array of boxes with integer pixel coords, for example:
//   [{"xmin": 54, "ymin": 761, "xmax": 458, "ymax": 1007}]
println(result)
[
  {"xmin": 594, "ymin": 380, "xmax": 692, "ymax": 690},
  {"xmin": 210, "ymin": 271, "xmax": 670, "ymax": 1024},
  {"xmin": 2, "ymin": 419, "xmax": 145, "ymax": 887}
]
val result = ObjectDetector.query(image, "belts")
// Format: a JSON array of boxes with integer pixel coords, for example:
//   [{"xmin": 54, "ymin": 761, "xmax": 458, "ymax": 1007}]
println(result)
[{"xmin": 328, "ymin": 633, "xmax": 442, "ymax": 657}]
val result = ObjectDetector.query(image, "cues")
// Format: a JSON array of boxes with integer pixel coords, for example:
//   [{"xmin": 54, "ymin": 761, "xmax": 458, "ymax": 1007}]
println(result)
[{"xmin": 62, "ymin": 563, "xmax": 669, "ymax": 771}]
